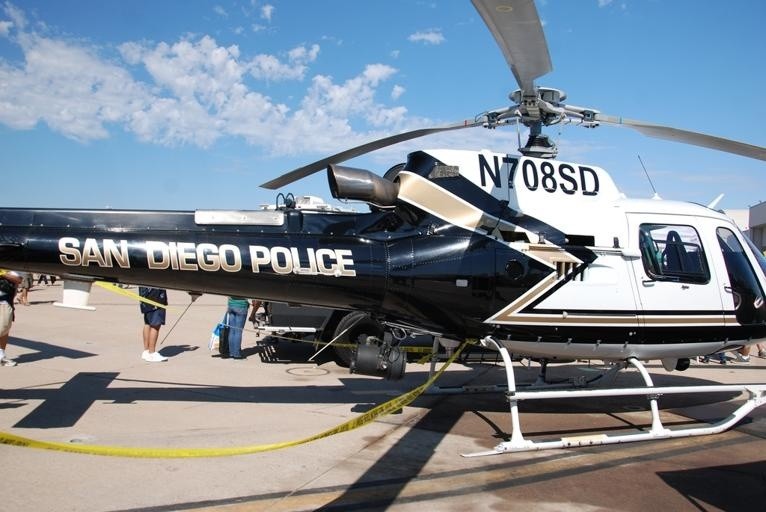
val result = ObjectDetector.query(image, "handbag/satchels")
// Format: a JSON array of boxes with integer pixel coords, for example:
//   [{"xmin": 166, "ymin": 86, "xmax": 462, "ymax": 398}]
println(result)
[{"xmin": 209, "ymin": 323, "xmax": 228, "ymax": 357}]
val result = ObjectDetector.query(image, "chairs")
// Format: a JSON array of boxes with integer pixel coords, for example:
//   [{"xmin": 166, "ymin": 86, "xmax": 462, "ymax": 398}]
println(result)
[{"xmin": 639, "ymin": 228, "xmax": 701, "ymax": 273}]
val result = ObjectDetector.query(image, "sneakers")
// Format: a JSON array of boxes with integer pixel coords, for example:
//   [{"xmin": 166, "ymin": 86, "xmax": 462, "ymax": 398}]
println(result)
[
  {"xmin": 1, "ymin": 357, "xmax": 16, "ymax": 366},
  {"xmin": 142, "ymin": 350, "xmax": 167, "ymax": 362},
  {"xmin": 230, "ymin": 354, "xmax": 247, "ymax": 359}
]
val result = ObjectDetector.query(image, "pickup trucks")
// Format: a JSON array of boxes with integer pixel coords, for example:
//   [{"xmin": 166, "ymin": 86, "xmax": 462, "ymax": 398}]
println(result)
[{"xmin": 257, "ymin": 301, "xmax": 577, "ymax": 368}]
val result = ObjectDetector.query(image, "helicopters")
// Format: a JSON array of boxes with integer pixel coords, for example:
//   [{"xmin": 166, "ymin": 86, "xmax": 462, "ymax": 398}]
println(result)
[{"xmin": 0, "ymin": 0, "xmax": 766, "ymax": 458}]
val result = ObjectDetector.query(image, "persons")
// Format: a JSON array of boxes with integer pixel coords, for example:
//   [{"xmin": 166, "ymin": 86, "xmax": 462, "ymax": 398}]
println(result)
[
  {"xmin": 249, "ymin": 299, "xmax": 261, "ymax": 322},
  {"xmin": 15, "ymin": 270, "xmax": 57, "ymax": 306},
  {"xmin": 737, "ymin": 341, "xmax": 766, "ymax": 361},
  {"xmin": 0, "ymin": 267, "xmax": 24, "ymax": 367},
  {"xmin": 701, "ymin": 352, "xmax": 726, "ymax": 364},
  {"xmin": 227, "ymin": 296, "xmax": 249, "ymax": 359},
  {"xmin": 139, "ymin": 286, "xmax": 168, "ymax": 363}
]
